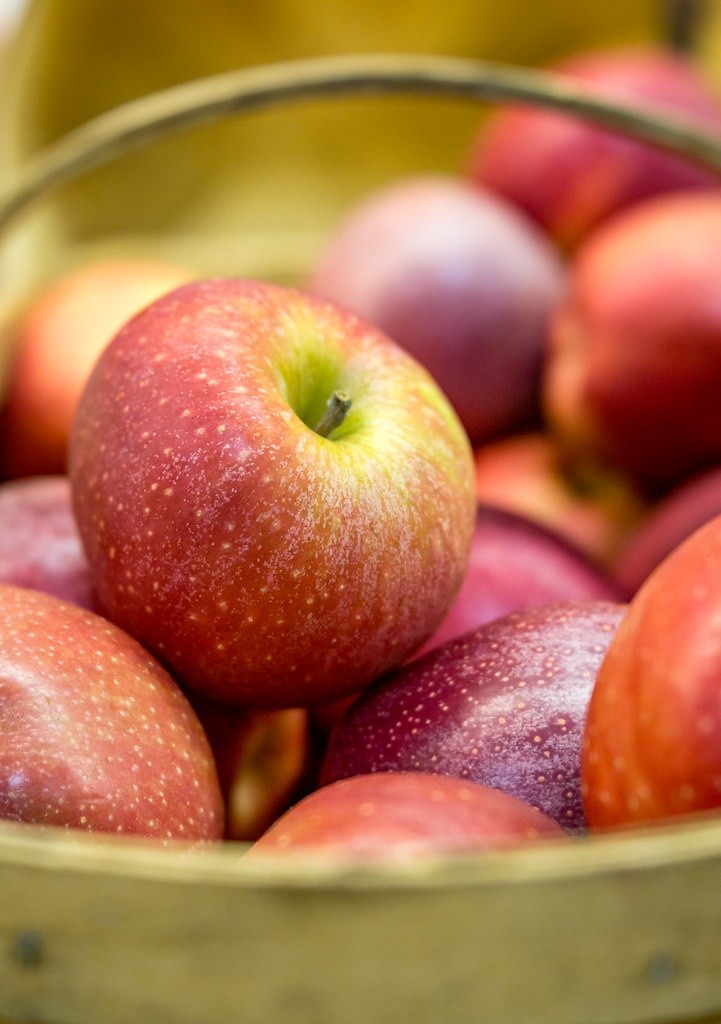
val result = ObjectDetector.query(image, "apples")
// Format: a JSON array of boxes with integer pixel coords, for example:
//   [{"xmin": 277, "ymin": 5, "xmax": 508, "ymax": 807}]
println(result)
[{"xmin": 2, "ymin": 44, "xmax": 721, "ymax": 859}]
